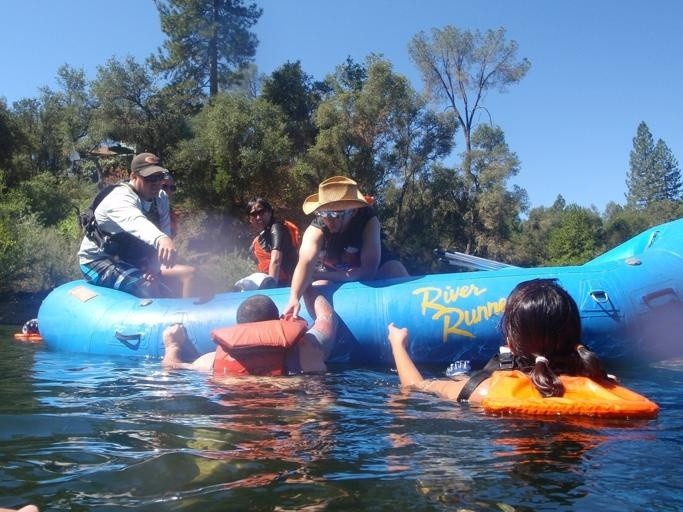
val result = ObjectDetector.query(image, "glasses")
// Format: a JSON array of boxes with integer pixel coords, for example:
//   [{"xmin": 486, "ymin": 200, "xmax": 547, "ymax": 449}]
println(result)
[
  {"xmin": 250, "ymin": 210, "xmax": 266, "ymax": 216},
  {"xmin": 320, "ymin": 211, "xmax": 351, "ymax": 218},
  {"xmin": 146, "ymin": 176, "xmax": 163, "ymax": 183}
]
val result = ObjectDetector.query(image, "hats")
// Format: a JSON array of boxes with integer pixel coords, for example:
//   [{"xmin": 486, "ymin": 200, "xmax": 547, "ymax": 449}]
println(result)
[
  {"xmin": 131, "ymin": 153, "xmax": 169, "ymax": 177},
  {"xmin": 303, "ymin": 176, "xmax": 369, "ymax": 216}
]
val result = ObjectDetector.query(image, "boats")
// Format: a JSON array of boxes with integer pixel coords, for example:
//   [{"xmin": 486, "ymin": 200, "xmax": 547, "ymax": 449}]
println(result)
[{"xmin": 34, "ymin": 219, "xmax": 683, "ymax": 371}]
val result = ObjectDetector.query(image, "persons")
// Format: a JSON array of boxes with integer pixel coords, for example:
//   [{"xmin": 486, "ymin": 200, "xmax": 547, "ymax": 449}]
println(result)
[
  {"xmin": 246, "ymin": 198, "xmax": 298, "ymax": 288},
  {"xmin": 282, "ymin": 177, "xmax": 411, "ymax": 319},
  {"xmin": 160, "ymin": 176, "xmax": 196, "ymax": 299},
  {"xmin": 22, "ymin": 319, "xmax": 38, "ymax": 334},
  {"xmin": 387, "ymin": 279, "xmax": 660, "ymax": 416},
  {"xmin": 77, "ymin": 152, "xmax": 177, "ymax": 299},
  {"xmin": 163, "ymin": 286, "xmax": 340, "ymax": 378}
]
